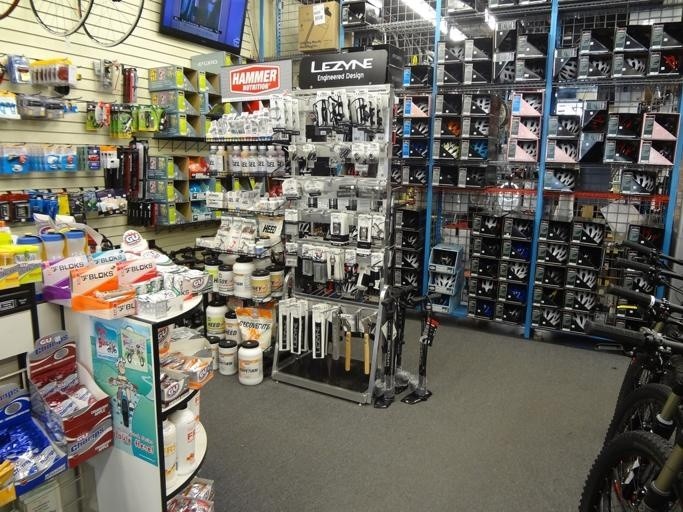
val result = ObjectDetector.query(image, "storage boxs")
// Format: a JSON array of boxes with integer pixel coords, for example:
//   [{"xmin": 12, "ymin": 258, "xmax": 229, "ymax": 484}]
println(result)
[
  {"xmin": 298, "ymin": 1, "xmax": 340, "ymax": 53},
  {"xmin": 146, "ymin": 51, "xmax": 270, "ymax": 227}
]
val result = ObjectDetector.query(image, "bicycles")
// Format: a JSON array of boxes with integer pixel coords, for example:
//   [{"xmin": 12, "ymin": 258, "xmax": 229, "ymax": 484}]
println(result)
[{"xmin": 576, "ymin": 241, "xmax": 682, "ymax": 508}]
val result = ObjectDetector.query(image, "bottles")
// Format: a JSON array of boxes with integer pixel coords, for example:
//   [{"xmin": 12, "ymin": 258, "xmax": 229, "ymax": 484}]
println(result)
[
  {"xmin": 207, "ymin": 145, "xmax": 287, "ymax": 176},
  {"xmin": 17, "ymin": 230, "xmax": 85, "ymax": 260},
  {"xmin": 259, "ymin": 196, "xmax": 284, "ymax": 210},
  {"xmin": 255, "ymin": 241, "xmax": 263, "ymax": 255}
]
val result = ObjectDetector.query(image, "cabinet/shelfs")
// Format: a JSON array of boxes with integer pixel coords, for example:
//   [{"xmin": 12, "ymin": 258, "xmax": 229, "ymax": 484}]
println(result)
[
  {"xmin": 394, "ymin": 0, "xmax": 683, "ymax": 355},
  {"xmin": 193, "ymin": 83, "xmax": 396, "ymax": 406},
  {"xmin": 47, "ymin": 263, "xmax": 209, "ymax": 512}
]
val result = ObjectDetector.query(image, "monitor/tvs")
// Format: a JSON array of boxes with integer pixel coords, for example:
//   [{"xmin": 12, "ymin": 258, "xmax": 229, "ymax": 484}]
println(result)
[{"xmin": 154, "ymin": 0, "xmax": 248, "ymax": 58}]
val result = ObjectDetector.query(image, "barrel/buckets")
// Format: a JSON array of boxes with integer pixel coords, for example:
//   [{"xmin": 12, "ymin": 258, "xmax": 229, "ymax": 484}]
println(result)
[
  {"xmin": 163, "ymin": 391, "xmax": 200, "ymax": 491},
  {"xmin": 204, "ymin": 255, "xmax": 284, "ymax": 385}
]
